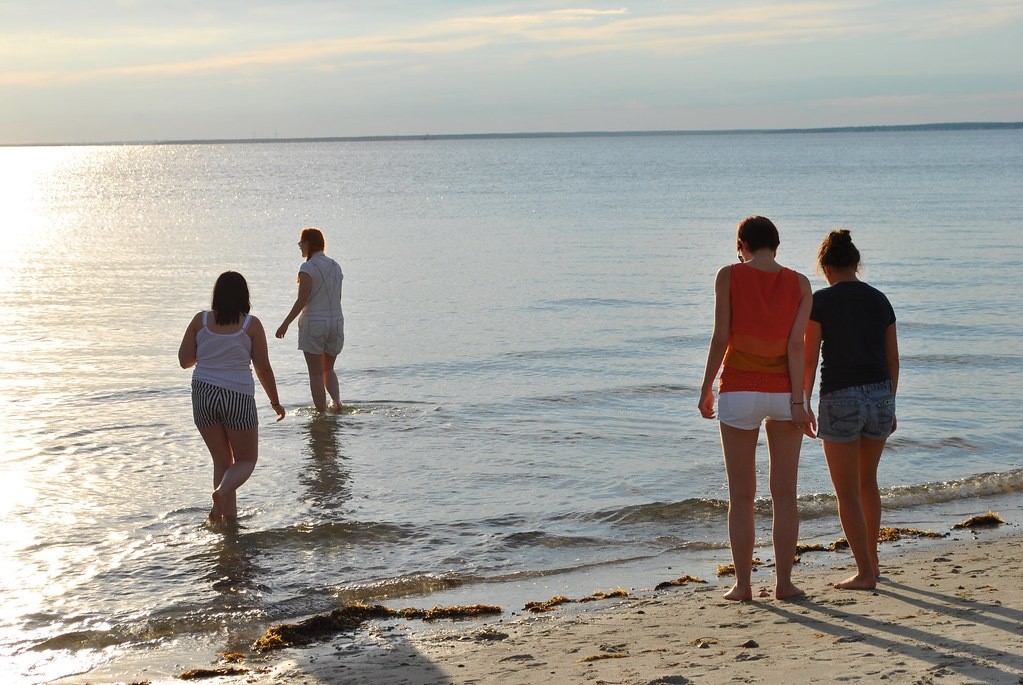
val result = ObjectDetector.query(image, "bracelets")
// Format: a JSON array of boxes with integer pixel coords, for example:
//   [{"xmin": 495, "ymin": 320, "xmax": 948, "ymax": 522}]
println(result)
[
  {"xmin": 270, "ymin": 401, "xmax": 279, "ymax": 406},
  {"xmin": 791, "ymin": 400, "xmax": 804, "ymax": 404}
]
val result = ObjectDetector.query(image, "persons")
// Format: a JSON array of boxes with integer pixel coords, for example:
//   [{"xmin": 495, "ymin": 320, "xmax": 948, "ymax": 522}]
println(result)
[
  {"xmin": 275, "ymin": 228, "xmax": 343, "ymax": 409},
  {"xmin": 804, "ymin": 229, "xmax": 899, "ymax": 588},
  {"xmin": 177, "ymin": 271, "xmax": 285, "ymax": 521},
  {"xmin": 697, "ymin": 217, "xmax": 812, "ymax": 602}
]
